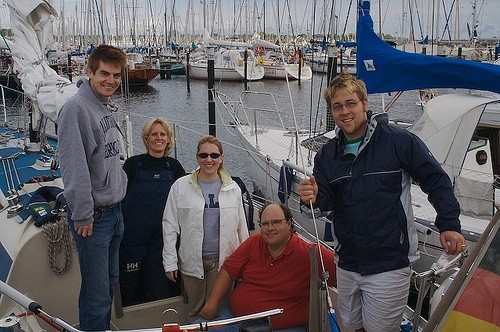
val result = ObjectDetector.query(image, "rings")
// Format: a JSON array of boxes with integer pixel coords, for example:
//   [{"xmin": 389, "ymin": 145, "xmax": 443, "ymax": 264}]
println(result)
[{"xmin": 461, "ymin": 244, "xmax": 466, "ymax": 246}]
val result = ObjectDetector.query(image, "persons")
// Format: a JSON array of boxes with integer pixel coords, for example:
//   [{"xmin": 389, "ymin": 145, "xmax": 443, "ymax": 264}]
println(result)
[
  {"xmin": 117, "ymin": 116, "xmax": 186, "ymax": 308},
  {"xmin": 298, "ymin": 73, "xmax": 467, "ymax": 331},
  {"xmin": 57, "ymin": 44, "xmax": 129, "ymax": 332},
  {"xmin": 162, "ymin": 135, "xmax": 250, "ymax": 323},
  {"xmin": 191, "ymin": 202, "xmax": 337, "ymax": 331}
]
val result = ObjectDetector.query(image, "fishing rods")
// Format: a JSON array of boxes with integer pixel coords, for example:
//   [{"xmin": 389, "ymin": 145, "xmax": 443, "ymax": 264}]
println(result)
[
  {"xmin": 271, "ymin": 0, "xmax": 340, "ymax": 332},
  {"xmin": 0, "ymin": 280, "xmax": 87, "ymax": 332}
]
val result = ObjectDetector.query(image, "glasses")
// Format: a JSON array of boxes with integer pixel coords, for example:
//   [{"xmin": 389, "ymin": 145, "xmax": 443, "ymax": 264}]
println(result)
[
  {"xmin": 260, "ymin": 218, "xmax": 287, "ymax": 229},
  {"xmin": 197, "ymin": 152, "xmax": 221, "ymax": 159},
  {"xmin": 329, "ymin": 98, "xmax": 363, "ymax": 112}
]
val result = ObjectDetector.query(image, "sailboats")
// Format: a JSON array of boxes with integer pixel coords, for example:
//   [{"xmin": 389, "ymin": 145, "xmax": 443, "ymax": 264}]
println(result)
[{"xmin": 0, "ymin": 1, "xmax": 500, "ymax": 332}]
